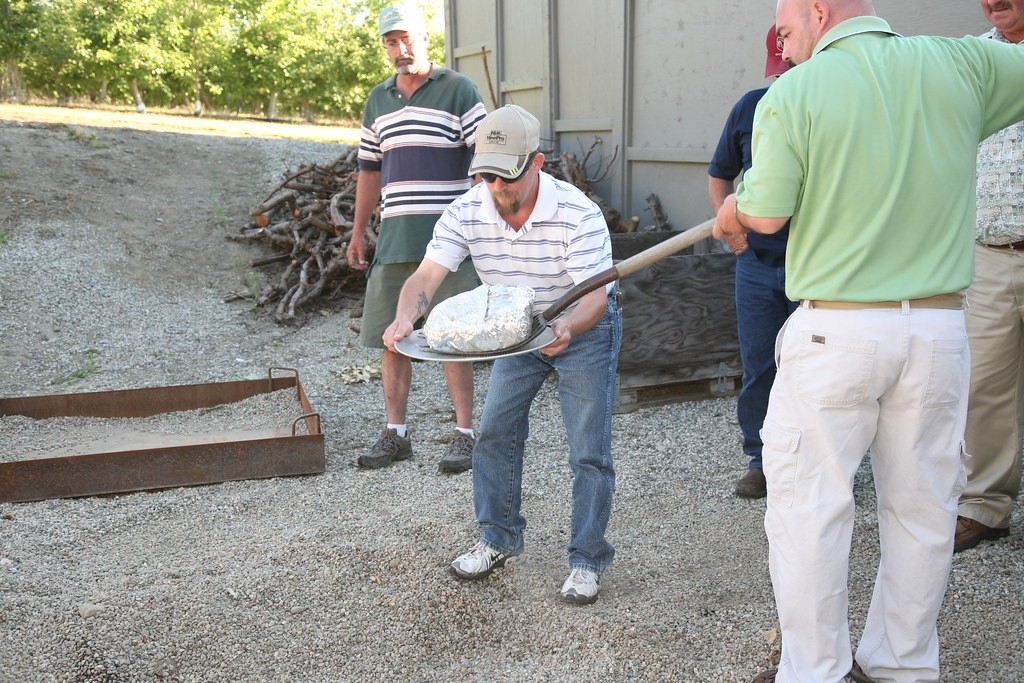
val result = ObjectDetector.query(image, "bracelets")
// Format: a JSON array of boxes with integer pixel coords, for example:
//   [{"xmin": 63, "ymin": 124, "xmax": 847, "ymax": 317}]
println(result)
[{"xmin": 736, "ymin": 206, "xmax": 749, "ymax": 230}]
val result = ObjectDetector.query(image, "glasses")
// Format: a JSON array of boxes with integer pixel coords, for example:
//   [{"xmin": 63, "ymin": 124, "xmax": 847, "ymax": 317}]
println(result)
[
  {"xmin": 383, "ymin": 36, "xmax": 424, "ymax": 52},
  {"xmin": 479, "ymin": 154, "xmax": 534, "ymax": 183}
]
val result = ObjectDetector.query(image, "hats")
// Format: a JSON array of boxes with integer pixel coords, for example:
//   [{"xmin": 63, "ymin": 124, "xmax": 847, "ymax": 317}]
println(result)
[
  {"xmin": 765, "ymin": 23, "xmax": 790, "ymax": 77},
  {"xmin": 376, "ymin": 2, "xmax": 426, "ymax": 41},
  {"xmin": 467, "ymin": 104, "xmax": 540, "ymax": 179}
]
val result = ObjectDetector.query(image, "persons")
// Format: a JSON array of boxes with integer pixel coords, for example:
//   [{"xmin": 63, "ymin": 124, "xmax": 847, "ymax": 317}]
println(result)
[
  {"xmin": 713, "ymin": 0, "xmax": 1024, "ymax": 683},
  {"xmin": 384, "ymin": 104, "xmax": 623, "ymax": 602},
  {"xmin": 346, "ymin": 2, "xmax": 489, "ymax": 474},
  {"xmin": 952, "ymin": 0, "xmax": 1024, "ymax": 554},
  {"xmin": 707, "ymin": 22, "xmax": 800, "ymax": 499}
]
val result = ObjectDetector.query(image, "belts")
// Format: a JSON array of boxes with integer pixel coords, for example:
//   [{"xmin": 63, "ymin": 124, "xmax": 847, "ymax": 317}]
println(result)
[
  {"xmin": 998, "ymin": 240, "xmax": 1024, "ymax": 251},
  {"xmin": 798, "ymin": 293, "xmax": 966, "ymax": 311}
]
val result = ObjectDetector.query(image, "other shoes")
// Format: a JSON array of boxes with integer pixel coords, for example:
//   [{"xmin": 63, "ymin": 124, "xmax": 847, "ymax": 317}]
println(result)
[
  {"xmin": 754, "ymin": 668, "xmax": 777, "ymax": 683},
  {"xmin": 847, "ymin": 649, "xmax": 873, "ymax": 683}
]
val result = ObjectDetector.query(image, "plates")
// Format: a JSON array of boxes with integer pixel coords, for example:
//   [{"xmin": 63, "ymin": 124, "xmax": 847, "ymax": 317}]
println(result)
[{"xmin": 395, "ymin": 329, "xmax": 558, "ymax": 361}]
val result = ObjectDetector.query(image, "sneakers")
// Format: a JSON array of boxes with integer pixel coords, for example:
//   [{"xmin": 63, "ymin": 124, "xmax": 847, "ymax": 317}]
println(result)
[
  {"xmin": 952, "ymin": 516, "xmax": 1011, "ymax": 553},
  {"xmin": 449, "ymin": 540, "xmax": 524, "ymax": 580},
  {"xmin": 440, "ymin": 430, "xmax": 477, "ymax": 473},
  {"xmin": 358, "ymin": 428, "xmax": 413, "ymax": 468},
  {"xmin": 561, "ymin": 566, "xmax": 599, "ymax": 603},
  {"xmin": 736, "ymin": 466, "xmax": 767, "ymax": 497}
]
